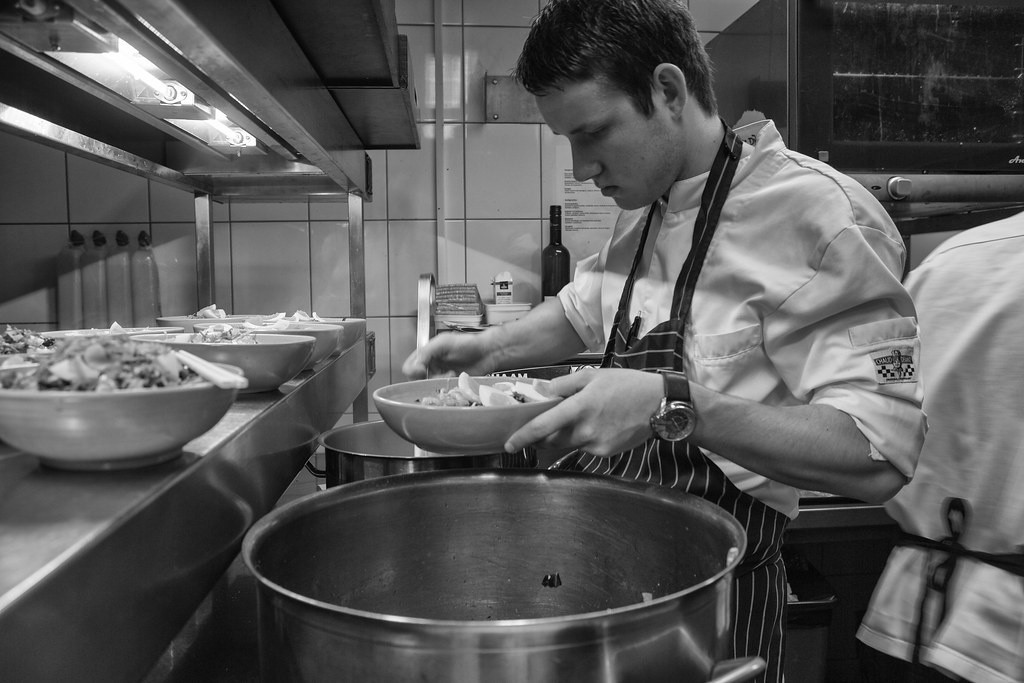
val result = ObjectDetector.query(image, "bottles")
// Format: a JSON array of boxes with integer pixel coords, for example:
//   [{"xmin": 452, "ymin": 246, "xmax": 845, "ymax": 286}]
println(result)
[
  {"xmin": 108, "ymin": 231, "xmax": 133, "ymax": 327},
  {"xmin": 81, "ymin": 229, "xmax": 109, "ymax": 328},
  {"xmin": 54, "ymin": 231, "xmax": 85, "ymax": 328},
  {"xmin": 131, "ymin": 231, "xmax": 160, "ymax": 327},
  {"xmin": 542, "ymin": 204, "xmax": 570, "ymax": 296}
]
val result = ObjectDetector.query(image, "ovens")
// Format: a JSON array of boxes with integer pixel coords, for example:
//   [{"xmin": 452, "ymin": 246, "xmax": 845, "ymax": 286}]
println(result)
[{"xmin": 699, "ymin": 0, "xmax": 1024, "ymax": 203}]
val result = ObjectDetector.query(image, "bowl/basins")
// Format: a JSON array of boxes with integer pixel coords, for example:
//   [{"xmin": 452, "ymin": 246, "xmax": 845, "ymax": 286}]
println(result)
[
  {"xmin": 156, "ymin": 315, "xmax": 269, "ymax": 334},
  {"xmin": 192, "ymin": 323, "xmax": 345, "ymax": 369},
  {"xmin": 38, "ymin": 328, "xmax": 184, "ymax": 343},
  {"xmin": 133, "ymin": 334, "xmax": 315, "ymax": 393},
  {"xmin": 283, "ymin": 317, "xmax": 367, "ymax": 349},
  {"xmin": 373, "ymin": 378, "xmax": 566, "ymax": 455},
  {"xmin": 0, "ymin": 363, "xmax": 244, "ymax": 470}
]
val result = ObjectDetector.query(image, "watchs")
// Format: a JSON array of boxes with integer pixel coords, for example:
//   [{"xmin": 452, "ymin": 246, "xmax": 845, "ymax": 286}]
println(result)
[{"xmin": 650, "ymin": 368, "xmax": 696, "ymax": 444}]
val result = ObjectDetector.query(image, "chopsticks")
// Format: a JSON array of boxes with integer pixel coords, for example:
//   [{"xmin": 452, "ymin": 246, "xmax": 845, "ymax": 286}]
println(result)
[{"xmin": 173, "ymin": 350, "xmax": 250, "ymax": 389}]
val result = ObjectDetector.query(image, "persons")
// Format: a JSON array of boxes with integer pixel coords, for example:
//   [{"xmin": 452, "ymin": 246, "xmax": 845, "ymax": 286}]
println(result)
[
  {"xmin": 854, "ymin": 209, "xmax": 1024, "ymax": 683},
  {"xmin": 402, "ymin": 1, "xmax": 929, "ymax": 683}
]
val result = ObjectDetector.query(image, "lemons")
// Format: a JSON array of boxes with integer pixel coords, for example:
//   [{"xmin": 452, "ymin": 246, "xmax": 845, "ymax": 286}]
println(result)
[
  {"xmin": 479, "ymin": 385, "xmax": 519, "ymax": 406},
  {"xmin": 458, "ymin": 371, "xmax": 481, "ymax": 404}
]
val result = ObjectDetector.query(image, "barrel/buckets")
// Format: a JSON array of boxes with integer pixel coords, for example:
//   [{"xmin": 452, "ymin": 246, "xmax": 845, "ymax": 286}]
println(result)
[
  {"xmin": 307, "ymin": 419, "xmax": 541, "ymax": 488},
  {"xmin": 241, "ymin": 469, "xmax": 760, "ymax": 683}
]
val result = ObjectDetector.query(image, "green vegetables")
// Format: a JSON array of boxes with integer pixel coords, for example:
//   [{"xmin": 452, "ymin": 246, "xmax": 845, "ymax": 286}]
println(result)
[{"xmin": 0, "ymin": 341, "xmax": 190, "ymax": 391}]
